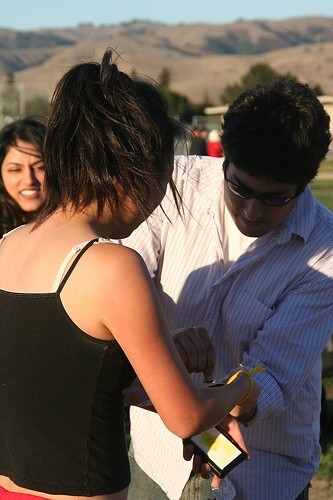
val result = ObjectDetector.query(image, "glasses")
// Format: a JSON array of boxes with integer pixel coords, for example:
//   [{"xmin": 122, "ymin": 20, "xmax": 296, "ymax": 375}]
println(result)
[{"xmin": 223, "ymin": 158, "xmax": 299, "ymax": 206}]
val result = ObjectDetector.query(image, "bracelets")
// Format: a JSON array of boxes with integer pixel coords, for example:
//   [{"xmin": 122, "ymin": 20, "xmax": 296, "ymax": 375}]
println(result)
[{"xmin": 226, "ymin": 363, "xmax": 265, "ymax": 406}]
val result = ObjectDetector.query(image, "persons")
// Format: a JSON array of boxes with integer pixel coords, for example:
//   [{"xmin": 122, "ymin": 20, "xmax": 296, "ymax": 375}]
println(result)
[
  {"xmin": 189, "ymin": 127, "xmax": 223, "ymax": 158},
  {"xmin": 0, "ymin": 116, "xmax": 57, "ymax": 239},
  {"xmin": 0, "ymin": 50, "xmax": 262, "ymax": 500},
  {"xmin": 98, "ymin": 80, "xmax": 333, "ymax": 500}
]
